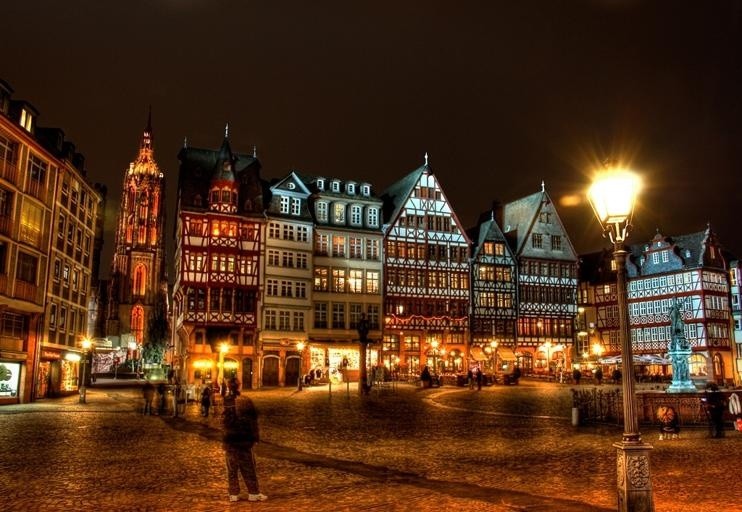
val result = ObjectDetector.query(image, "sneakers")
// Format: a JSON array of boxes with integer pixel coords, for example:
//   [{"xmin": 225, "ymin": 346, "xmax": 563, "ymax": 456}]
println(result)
[
  {"xmin": 229, "ymin": 494, "xmax": 239, "ymax": 501},
  {"xmin": 248, "ymin": 493, "xmax": 266, "ymax": 501}
]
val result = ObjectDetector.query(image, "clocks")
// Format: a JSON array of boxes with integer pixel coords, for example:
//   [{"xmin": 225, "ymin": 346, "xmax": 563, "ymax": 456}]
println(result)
[{"xmin": 656, "ymin": 405, "xmax": 676, "ymax": 424}]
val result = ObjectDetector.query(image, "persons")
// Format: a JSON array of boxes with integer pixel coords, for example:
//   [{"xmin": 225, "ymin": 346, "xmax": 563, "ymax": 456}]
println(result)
[
  {"xmin": 594, "ymin": 367, "xmax": 602, "ymax": 384},
  {"xmin": 667, "ymin": 296, "xmax": 691, "ymax": 350},
  {"xmin": 141, "ymin": 378, "xmax": 227, "ymax": 418},
  {"xmin": 476, "ymin": 367, "xmax": 482, "ymax": 390},
  {"xmin": 611, "ymin": 367, "xmax": 621, "ymax": 380},
  {"xmin": 221, "ymin": 377, "xmax": 269, "ymax": 502},
  {"xmin": 572, "ymin": 367, "xmax": 582, "ymax": 384},
  {"xmin": 467, "ymin": 368, "xmax": 474, "ymax": 389},
  {"xmin": 420, "ymin": 365, "xmax": 432, "ymax": 388},
  {"xmin": 704, "ymin": 381, "xmax": 727, "ymax": 438}
]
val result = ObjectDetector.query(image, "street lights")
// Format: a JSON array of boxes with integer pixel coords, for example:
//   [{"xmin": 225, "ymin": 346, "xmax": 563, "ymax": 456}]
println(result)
[
  {"xmin": 79, "ymin": 340, "xmax": 91, "ymax": 404},
  {"xmin": 430, "ymin": 341, "xmax": 439, "ymax": 374},
  {"xmin": 585, "ymin": 167, "xmax": 652, "ymax": 512},
  {"xmin": 491, "ymin": 341, "xmax": 499, "ymax": 375},
  {"xmin": 545, "ymin": 342, "xmax": 553, "ymax": 382}
]
